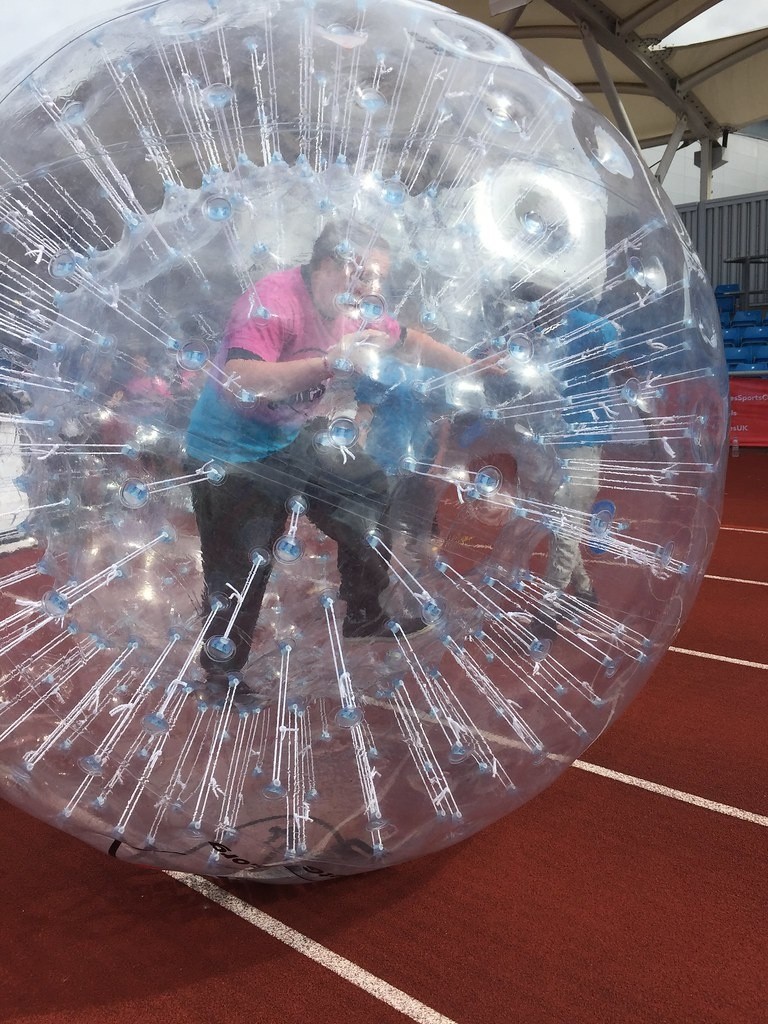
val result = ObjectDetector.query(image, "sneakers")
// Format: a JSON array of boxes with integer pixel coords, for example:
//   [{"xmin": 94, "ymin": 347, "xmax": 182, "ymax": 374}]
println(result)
[
  {"xmin": 554, "ymin": 589, "xmax": 599, "ymax": 620},
  {"xmin": 341, "ymin": 613, "xmax": 436, "ymax": 644},
  {"xmin": 203, "ymin": 676, "xmax": 275, "ymax": 714},
  {"xmin": 509, "ymin": 615, "xmax": 559, "ymax": 650}
]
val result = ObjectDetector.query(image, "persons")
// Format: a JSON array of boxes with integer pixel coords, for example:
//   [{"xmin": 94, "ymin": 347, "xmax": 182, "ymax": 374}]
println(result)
[
  {"xmin": 109, "ymin": 357, "xmax": 172, "ymax": 414},
  {"xmin": 347, "ymin": 339, "xmax": 457, "ymax": 622},
  {"xmin": 505, "ymin": 272, "xmax": 665, "ymax": 640},
  {"xmin": 182, "ymin": 220, "xmax": 508, "ymax": 700}
]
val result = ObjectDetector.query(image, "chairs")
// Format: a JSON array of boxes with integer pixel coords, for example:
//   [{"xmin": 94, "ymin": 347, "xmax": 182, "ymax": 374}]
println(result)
[{"xmin": 619, "ymin": 283, "xmax": 768, "ymax": 372}]
